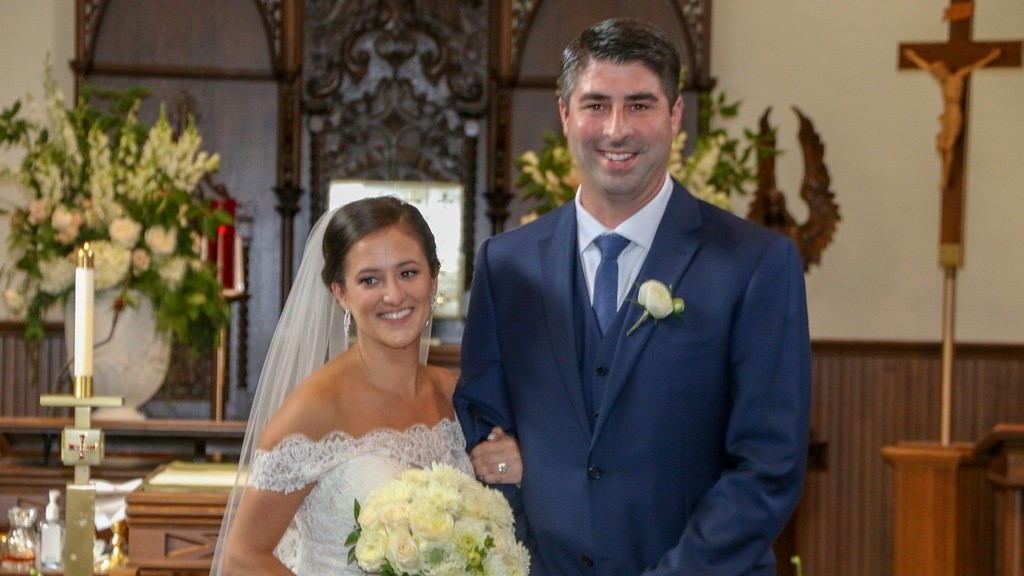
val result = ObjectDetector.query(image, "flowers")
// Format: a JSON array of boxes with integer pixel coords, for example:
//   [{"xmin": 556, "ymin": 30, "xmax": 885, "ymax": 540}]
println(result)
[
  {"xmin": 344, "ymin": 460, "xmax": 532, "ymax": 576},
  {"xmin": 623, "ymin": 278, "xmax": 689, "ymax": 339},
  {"xmin": 514, "ymin": 66, "xmax": 761, "ymax": 221},
  {"xmin": 0, "ymin": 44, "xmax": 220, "ymax": 322}
]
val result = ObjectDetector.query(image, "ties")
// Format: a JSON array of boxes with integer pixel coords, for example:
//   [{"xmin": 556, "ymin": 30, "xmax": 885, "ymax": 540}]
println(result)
[{"xmin": 593, "ymin": 234, "xmax": 631, "ymax": 338}]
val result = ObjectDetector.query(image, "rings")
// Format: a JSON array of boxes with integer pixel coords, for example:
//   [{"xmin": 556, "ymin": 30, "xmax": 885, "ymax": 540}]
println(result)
[{"xmin": 498, "ymin": 462, "xmax": 507, "ymax": 474}]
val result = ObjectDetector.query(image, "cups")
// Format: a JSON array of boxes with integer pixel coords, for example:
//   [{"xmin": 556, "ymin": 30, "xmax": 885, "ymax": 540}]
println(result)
[{"xmin": 2, "ymin": 508, "xmax": 37, "ymax": 574}]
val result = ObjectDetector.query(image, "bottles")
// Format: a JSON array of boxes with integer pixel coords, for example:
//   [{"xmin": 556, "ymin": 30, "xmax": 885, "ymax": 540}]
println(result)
[{"xmin": 38, "ymin": 489, "xmax": 65, "ymax": 572}]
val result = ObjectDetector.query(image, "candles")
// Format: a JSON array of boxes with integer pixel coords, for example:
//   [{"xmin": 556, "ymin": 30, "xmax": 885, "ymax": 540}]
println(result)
[{"xmin": 72, "ymin": 242, "xmax": 95, "ymax": 380}]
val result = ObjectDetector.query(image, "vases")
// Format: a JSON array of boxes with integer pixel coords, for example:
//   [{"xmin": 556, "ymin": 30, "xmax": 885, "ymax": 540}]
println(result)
[{"xmin": 63, "ymin": 287, "xmax": 173, "ymax": 421}]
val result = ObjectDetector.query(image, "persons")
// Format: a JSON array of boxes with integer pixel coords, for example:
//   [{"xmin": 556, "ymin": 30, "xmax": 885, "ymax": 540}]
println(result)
[
  {"xmin": 215, "ymin": 195, "xmax": 523, "ymax": 575},
  {"xmin": 453, "ymin": 16, "xmax": 812, "ymax": 576},
  {"xmin": 904, "ymin": 46, "xmax": 1002, "ymax": 189}
]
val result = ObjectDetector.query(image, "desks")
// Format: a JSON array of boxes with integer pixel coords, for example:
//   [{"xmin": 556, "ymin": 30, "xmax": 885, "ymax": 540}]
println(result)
[{"xmin": 125, "ymin": 459, "xmax": 238, "ymax": 576}]
